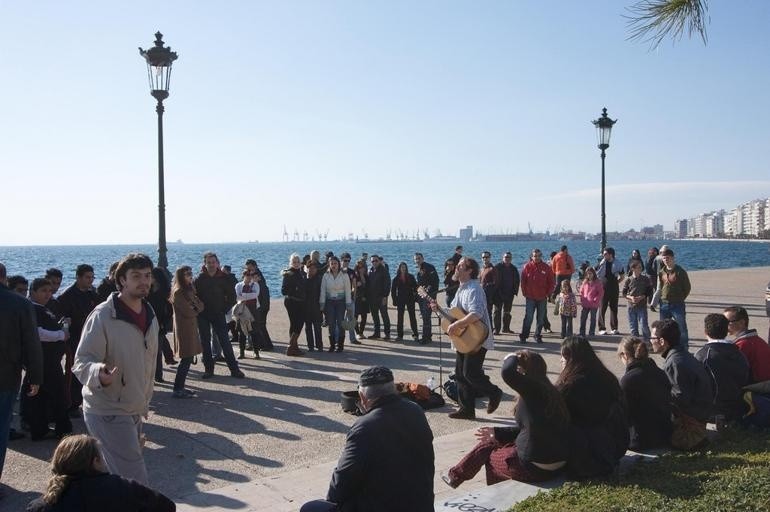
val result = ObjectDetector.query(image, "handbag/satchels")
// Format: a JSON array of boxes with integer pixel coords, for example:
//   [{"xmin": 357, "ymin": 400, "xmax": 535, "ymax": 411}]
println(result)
[
  {"xmin": 565, "ymin": 262, "xmax": 570, "ymax": 270},
  {"xmin": 735, "ymin": 379, "xmax": 770, "ymax": 428},
  {"xmin": 340, "ymin": 314, "xmax": 355, "ymax": 330}
]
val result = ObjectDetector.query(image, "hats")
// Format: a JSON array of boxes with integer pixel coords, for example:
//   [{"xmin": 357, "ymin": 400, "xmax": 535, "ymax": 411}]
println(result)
[{"xmin": 355, "ymin": 366, "xmax": 395, "ymax": 389}]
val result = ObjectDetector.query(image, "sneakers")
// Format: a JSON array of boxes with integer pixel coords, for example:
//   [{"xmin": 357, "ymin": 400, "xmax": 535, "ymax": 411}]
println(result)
[
  {"xmin": 172, "ymin": 390, "xmax": 192, "ymax": 399},
  {"xmin": 448, "ymin": 408, "xmax": 477, "ymax": 421},
  {"xmin": 231, "ymin": 369, "xmax": 245, "ymax": 377},
  {"xmin": 489, "ymin": 328, "xmax": 622, "ymax": 345},
  {"xmin": 439, "ymin": 466, "xmax": 458, "ymax": 489},
  {"xmin": 287, "ymin": 328, "xmax": 431, "ymax": 356},
  {"xmin": 202, "ymin": 371, "xmax": 214, "ymax": 379},
  {"xmin": 165, "ymin": 359, "xmax": 179, "ymax": 364},
  {"xmin": 182, "ymin": 388, "xmax": 192, "ymax": 394},
  {"xmin": 8, "ymin": 407, "xmax": 81, "ymax": 442},
  {"xmin": 486, "ymin": 387, "xmax": 502, "ymax": 415},
  {"xmin": 210, "ymin": 336, "xmax": 276, "ymax": 362}
]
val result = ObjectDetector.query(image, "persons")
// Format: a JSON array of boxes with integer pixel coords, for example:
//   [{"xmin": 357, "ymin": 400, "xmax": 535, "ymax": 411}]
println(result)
[
  {"xmin": 149, "ymin": 253, "xmax": 247, "ymax": 398},
  {"xmin": 443, "ymin": 245, "xmax": 465, "ymax": 305},
  {"xmin": 222, "ymin": 259, "xmax": 272, "ymax": 360},
  {"xmin": 696, "ymin": 314, "xmax": 750, "ymax": 423},
  {"xmin": 615, "ymin": 335, "xmax": 673, "ymax": 456},
  {"xmin": 73, "ymin": 251, "xmax": 161, "ymax": 488},
  {"xmin": 429, "ymin": 257, "xmax": 502, "ymax": 420},
  {"xmin": 300, "ymin": 366, "xmax": 435, "ymax": 511},
  {"xmin": 519, "ymin": 249, "xmax": 554, "ymax": 345},
  {"xmin": 25, "ymin": 435, "xmax": 177, "ymax": 511},
  {"xmin": 649, "ymin": 318, "xmax": 718, "ymax": 424},
  {"xmin": 439, "ymin": 352, "xmax": 568, "ymax": 488},
  {"xmin": 280, "ymin": 247, "xmax": 391, "ymax": 357},
  {"xmin": 533, "ymin": 245, "xmax": 577, "ymax": 338},
  {"xmin": 1, "ymin": 261, "xmax": 117, "ymax": 482},
  {"xmin": 390, "ymin": 253, "xmax": 439, "ymax": 345},
  {"xmin": 480, "ymin": 251, "xmax": 519, "ymax": 335},
  {"xmin": 723, "ymin": 305, "xmax": 770, "ymax": 418},
  {"xmin": 556, "ymin": 333, "xmax": 631, "ymax": 471},
  {"xmin": 578, "ymin": 245, "xmax": 691, "ymax": 344}
]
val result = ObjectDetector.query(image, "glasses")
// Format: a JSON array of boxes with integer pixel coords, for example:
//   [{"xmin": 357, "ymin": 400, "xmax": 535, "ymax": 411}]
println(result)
[
  {"xmin": 647, "ymin": 336, "xmax": 658, "ymax": 341},
  {"xmin": 184, "ymin": 272, "xmax": 192, "ymax": 276},
  {"xmin": 603, "ymin": 253, "xmax": 608, "ymax": 257},
  {"xmin": 727, "ymin": 319, "xmax": 740, "ymax": 323},
  {"xmin": 15, "ymin": 287, "xmax": 29, "ymax": 293},
  {"xmin": 85, "ymin": 275, "xmax": 95, "ymax": 280},
  {"xmin": 343, "ymin": 260, "xmax": 350, "ymax": 262}
]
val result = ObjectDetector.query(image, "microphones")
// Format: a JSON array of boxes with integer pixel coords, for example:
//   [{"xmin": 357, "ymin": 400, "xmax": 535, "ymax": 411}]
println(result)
[{"xmin": 438, "ymin": 280, "xmax": 456, "ymax": 293}]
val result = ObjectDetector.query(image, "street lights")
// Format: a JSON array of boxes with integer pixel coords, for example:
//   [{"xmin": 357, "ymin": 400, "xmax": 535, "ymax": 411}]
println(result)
[
  {"xmin": 139, "ymin": 26, "xmax": 178, "ymax": 268},
  {"xmin": 590, "ymin": 107, "xmax": 618, "ymax": 252}
]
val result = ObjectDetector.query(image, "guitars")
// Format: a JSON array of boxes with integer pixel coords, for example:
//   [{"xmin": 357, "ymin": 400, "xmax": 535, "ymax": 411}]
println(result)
[{"xmin": 416, "ymin": 285, "xmax": 489, "ymax": 355}]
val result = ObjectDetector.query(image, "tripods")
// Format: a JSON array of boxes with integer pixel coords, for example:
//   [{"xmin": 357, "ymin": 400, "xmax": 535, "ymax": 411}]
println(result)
[{"xmin": 427, "ymin": 295, "xmax": 450, "ymax": 399}]
